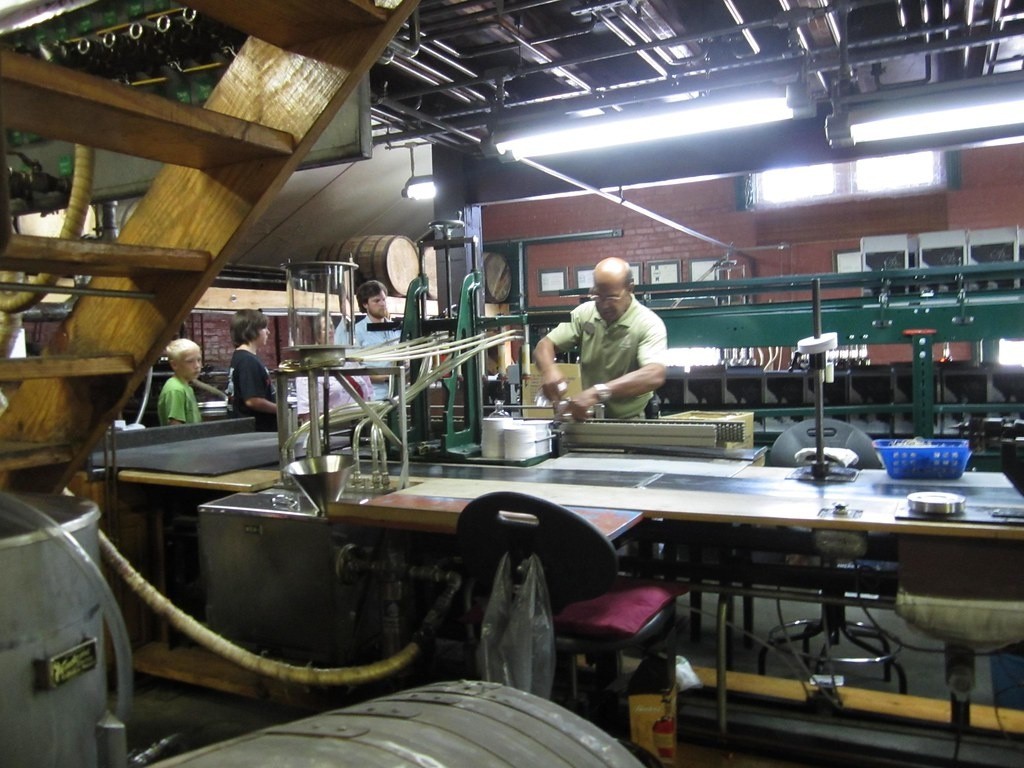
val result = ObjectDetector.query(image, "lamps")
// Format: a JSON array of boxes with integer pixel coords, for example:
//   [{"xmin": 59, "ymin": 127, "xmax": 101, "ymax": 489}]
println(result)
[
  {"xmin": 478, "ymin": 1, "xmax": 1024, "ymax": 166},
  {"xmin": 402, "ymin": 146, "xmax": 436, "ymax": 200}
]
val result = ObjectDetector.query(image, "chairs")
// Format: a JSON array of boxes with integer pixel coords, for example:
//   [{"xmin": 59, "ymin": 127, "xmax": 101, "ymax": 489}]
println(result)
[
  {"xmin": 756, "ymin": 419, "xmax": 909, "ymax": 696},
  {"xmin": 457, "ymin": 492, "xmax": 678, "ymax": 738}
]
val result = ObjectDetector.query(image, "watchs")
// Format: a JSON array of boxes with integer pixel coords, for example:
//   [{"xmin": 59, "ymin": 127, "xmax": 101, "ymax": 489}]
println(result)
[{"xmin": 595, "ymin": 384, "xmax": 612, "ymax": 401}]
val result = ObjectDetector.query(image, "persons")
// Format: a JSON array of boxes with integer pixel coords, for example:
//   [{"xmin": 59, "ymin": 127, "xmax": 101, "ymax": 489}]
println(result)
[
  {"xmin": 227, "ymin": 308, "xmax": 278, "ymax": 432},
  {"xmin": 354, "ymin": 280, "xmax": 410, "ymax": 401},
  {"xmin": 314, "ymin": 314, "xmax": 335, "ymax": 346},
  {"xmin": 532, "ymin": 257, "xmax": 668, "ymax": 655},
  {"xmin": 156, "ymin": 338, "xmax": 202, "ymax": 428}
]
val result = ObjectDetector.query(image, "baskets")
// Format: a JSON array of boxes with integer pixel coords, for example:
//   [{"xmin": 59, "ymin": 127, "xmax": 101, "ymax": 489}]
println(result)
[{"xmin": 873, "ymin": 438, "xmax": 970, "ymax": 481}]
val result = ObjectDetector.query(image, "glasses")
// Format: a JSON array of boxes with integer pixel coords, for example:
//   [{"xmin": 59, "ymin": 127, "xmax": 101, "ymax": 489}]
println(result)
[{"xmin": 587, "ymin": 286, "xmax": 628, "ymax": 303}]
